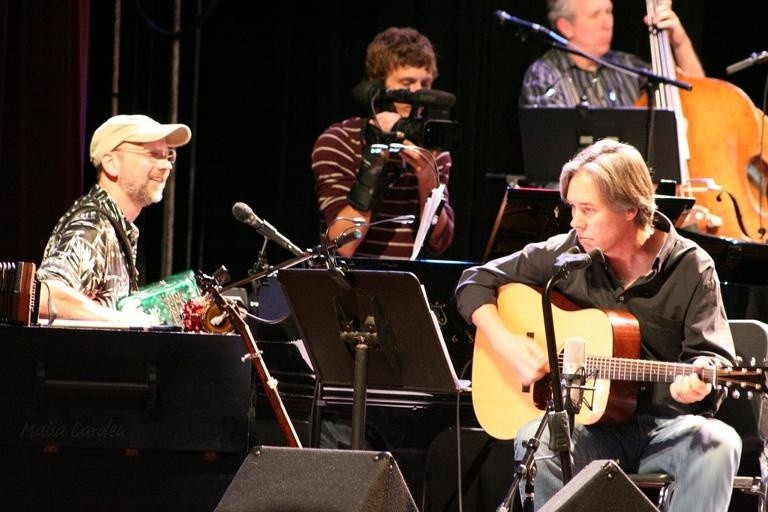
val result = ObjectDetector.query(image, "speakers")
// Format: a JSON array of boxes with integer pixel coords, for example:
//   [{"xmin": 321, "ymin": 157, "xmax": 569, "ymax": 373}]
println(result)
[
  {"xmin": 214, "ymin": 445, "xmax": 416, "ymax": 512},
  {"xmin": 529, "ymin": 459, "xmax": 660, "ymax": 512}
]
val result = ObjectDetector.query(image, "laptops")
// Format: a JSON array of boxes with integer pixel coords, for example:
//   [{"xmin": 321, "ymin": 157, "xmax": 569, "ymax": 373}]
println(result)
[{"xmin": 517, "ymin": 101, "xmax": 682, "ymax": 185}]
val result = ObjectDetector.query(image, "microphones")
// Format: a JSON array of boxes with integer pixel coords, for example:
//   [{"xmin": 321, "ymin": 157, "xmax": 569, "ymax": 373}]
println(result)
[
  {"xmin": 232, "ymin": 203, "xmax": 298, "ymax": 250},
  {"xmin": 497, "ymin": 9, "xmax": 541, "ymax": 28}
]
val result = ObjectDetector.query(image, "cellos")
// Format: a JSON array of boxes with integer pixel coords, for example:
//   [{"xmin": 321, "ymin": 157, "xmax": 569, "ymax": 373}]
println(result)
[{"xmin": 633, "ymin": 0, "xmax": 768, "ymax": 245}]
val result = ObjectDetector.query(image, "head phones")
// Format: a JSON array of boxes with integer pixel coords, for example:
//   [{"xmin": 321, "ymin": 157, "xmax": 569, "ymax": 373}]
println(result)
[{"xmin": 574, "ymin": 104, "xmax": 601, "ymax": 151}]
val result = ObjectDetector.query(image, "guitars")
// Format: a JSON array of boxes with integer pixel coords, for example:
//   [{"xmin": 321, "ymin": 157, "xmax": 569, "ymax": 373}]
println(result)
[{"xmin": 471, "ymin": 282, "xmax": 768, "ymax": 440}]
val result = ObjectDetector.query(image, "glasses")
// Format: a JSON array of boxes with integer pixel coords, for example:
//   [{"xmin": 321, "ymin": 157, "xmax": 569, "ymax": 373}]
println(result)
[{"xmin": 117, "ymin": 148, "xmax": 177, "ymax": 162}]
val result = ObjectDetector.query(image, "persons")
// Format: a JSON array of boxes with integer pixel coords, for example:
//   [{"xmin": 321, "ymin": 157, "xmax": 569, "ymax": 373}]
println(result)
[
  {"xmin": 518, "ymin": 0, "xmax": 706, "ymax": 190},
  {"xmin": 310, "ymin": 27, "xmax": 454, "ymax": 258},
  {"xmin": 37, "ymin": 113, "xmax": 195, "ymax": 321},
  {"xmin": 454, "ymin": 138, "xmax": 743, "ymax": 512}
]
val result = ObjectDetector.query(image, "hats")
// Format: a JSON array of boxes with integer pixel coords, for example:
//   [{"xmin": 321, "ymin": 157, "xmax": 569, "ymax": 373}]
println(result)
[{"xmin": 89, "ymin": 115, "xmax": 192, "ymax": 167}]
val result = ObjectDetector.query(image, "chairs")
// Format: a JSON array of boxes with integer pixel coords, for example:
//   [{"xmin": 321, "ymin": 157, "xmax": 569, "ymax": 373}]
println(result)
[{"xmin": 627, "ymin": 315, "xmax": 766, "ymax": 512}]
